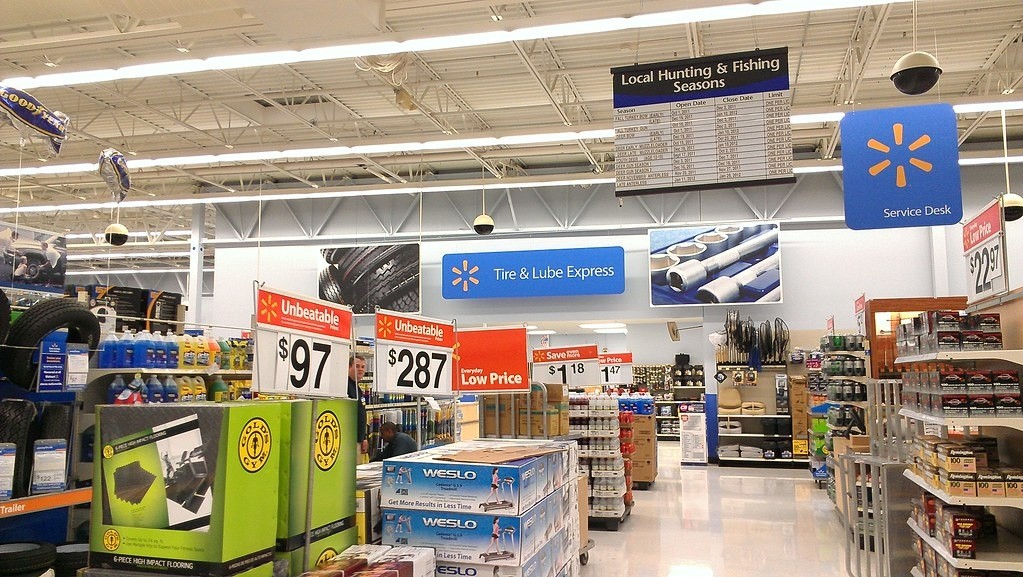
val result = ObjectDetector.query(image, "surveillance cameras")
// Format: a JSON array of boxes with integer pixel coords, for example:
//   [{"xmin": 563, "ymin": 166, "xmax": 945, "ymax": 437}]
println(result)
[
  {"xmin": 999, "ymin": 194, "xmax": 1023, "ymax": 221},
  {"xmin": 105, "ymin": 225, "xmax": 129, "ymax": 246},
  {"xmin": 890, "ymin": 50, "xmax": 943, "ymax": 96},
  {"xmin": 473, "ymin": 214, "xmax": 494, "ymax": 236}
]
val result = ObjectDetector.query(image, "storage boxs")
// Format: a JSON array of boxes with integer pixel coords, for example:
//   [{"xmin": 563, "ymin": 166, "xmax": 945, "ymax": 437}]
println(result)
[
  {"xmin": 79, "ymin": 382, "xmax": 589, "ymax": 577},
  {"xmin": 630, "ymin": 409, "xmax": 660, "ymax": 485},
  {"xmin": 784, "ymin": 307, "xmax": 1023, "ymax": 577}
]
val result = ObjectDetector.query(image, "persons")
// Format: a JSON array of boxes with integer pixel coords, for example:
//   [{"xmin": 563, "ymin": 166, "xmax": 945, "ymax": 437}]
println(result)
[
  {"xmin": 369, "ymin": 422, "xmax": 416, "ymax": 463},
  {"xmin": 14, "ymin": 256, "xmax": 30, "ymax": 283},
  {"xmin": 0, "ymin": 231, "xmax": 19, "ymax": 259},
  {"xmin": 38, "ymin": 236, "xmax": 64, "ymax": 287},
  {"xmin": 396, "ymin": 466, "xmax": 406, "ymax": 484},
  {"xmin": 485, "ymin": 466, "xmax": 502, "ymax": 505},
  {"xmin": 396, "ymin": 514, "xmax": 407, "ymax": 532},
  {"xmin": 347, "ymin": 355, "xmax": 368, "ymax": 465},
  {"xmin": 485, "ymin": 518, "xmax": 503, "ymax": 555}
]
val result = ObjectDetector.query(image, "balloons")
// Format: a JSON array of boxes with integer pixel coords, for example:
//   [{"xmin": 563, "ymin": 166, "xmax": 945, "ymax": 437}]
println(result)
[
  {"xmin": 98, "ymin": 148, "xmax": 131, "ymax": 203},
  {"xmin": 0, "ymin": 85, "xmax": 69, "ymax": 159}
]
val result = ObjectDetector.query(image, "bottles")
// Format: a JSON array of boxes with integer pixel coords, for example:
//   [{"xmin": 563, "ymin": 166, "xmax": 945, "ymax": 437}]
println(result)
[
  {"xmin": 568, "ymin": 365, "xmax": 666, "ymax": 510},
  {"xmin": 98, "ymin": 330, "xmax": 254, "ymax": 404},
  {"xmin": 820, "ymin": 332, "xmax": 864, "ymax": 426}
]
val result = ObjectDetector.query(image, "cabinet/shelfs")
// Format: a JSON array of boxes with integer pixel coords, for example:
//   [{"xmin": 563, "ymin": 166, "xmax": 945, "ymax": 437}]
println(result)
[
  {"xmin": 817, "ymin": 339, "xmax": 902, "ymax": 551},
  {"xmin": 894, "ymin": 287, "xmax": 1023, "ymax": 577},
  {"xmin": 68, "ymin": 332, "xmax": 253, "ymax": 546},
  {"xmin": 654, "ymin": 366, "xmax": 705, "ymax": 441},
  {"xmin": 784, "ymin": 358, "xmax": 810, "ymax": 470},
  {"xmin": 713, "ymin": 362, "xmax": 793, "ymax": 470},
  {"xmin": 0, "ymin": 304, "xmax": 92, "ymax": 577}
]
